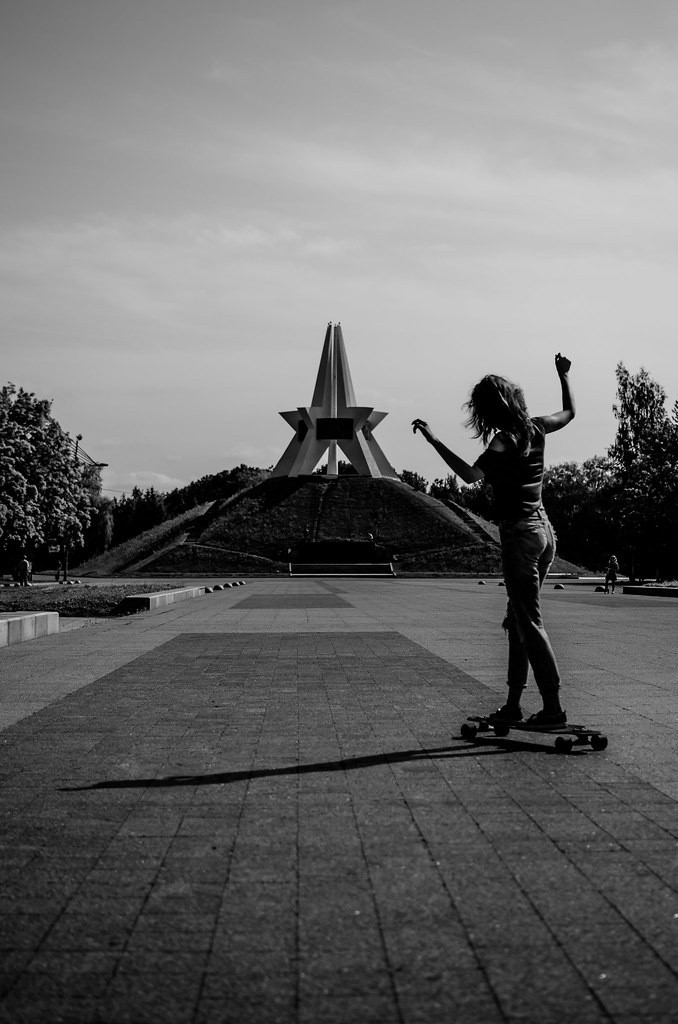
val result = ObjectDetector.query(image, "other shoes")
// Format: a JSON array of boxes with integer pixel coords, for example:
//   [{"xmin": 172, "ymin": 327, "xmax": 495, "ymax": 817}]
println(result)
[
  {"xmin": 485, "ymin": 703, "xmax": 523, "ymax": 725},
  {"xmin": 516, "ymin": 709, "xmax": 567, "ymax": 728},
  {"xmin": 612, "ymin": 591, "xmax": 615, "ymax": 594}
]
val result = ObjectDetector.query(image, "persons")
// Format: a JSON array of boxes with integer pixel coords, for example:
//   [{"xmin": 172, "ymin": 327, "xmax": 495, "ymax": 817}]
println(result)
[
  {"xmin": 412, "ymin": 352, "xmax": 575, "ymax": 729},
  {"xmin": 18, "ymin": 554, "xmax": 32, "ymax": 586},
  {"xmin": 603, "ymin": 556, "xmax": 619, "ymax": 594}
]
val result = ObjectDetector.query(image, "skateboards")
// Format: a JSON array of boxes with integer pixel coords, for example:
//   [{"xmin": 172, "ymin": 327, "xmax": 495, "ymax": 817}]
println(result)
[{"xmin": 460, "ymin": 714, "xmax": 608, "ymax": 754}]
